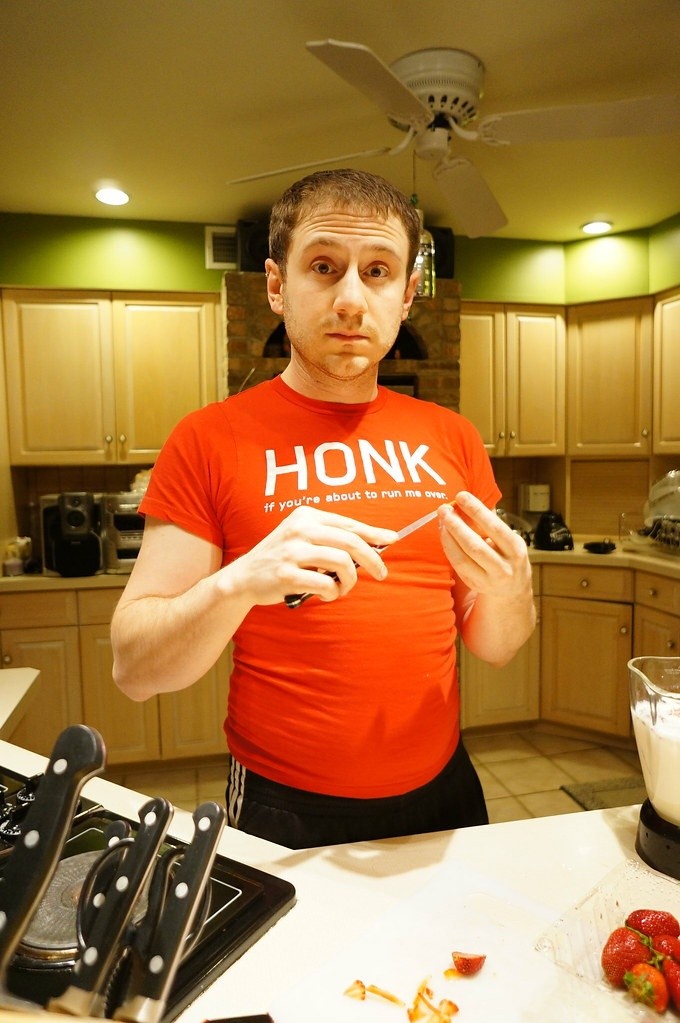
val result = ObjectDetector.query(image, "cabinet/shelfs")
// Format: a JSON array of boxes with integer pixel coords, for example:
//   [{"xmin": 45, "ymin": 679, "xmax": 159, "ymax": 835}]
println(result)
[
  {"xmin": 460, "ymin": 564, "xmax": 540, "ymax": 729},
  {"xmin": 457, "ymin": 300, "xmax": 566, "ymax": 457},
  {"xmin": 632, "ymin": 569, "xmax": 680, "ymax": 706},
  {"xmin": 0, "ymin": 588, "xmax": 236, "ymax": 765},
  {"xmin": 652, "ymin": 286, "xmax": 680, "ymax": 457},
  {"xmin": 540, "ymin": 564, "xmax": 636, "ymax": 738},
  {"xmin": 0, "ymin": 288, "xmax": 217, "ymax": 467},
  {"xmin": 566, "ymin": 295, "xmax": 654, "ymax": 459}
]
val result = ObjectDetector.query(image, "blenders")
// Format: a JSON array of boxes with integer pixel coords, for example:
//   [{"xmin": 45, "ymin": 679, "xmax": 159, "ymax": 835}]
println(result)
[
  {"xmin": 627, "ymin": 656, "xmax": 680, "ymax": 880},
  {"xmin": 533, "ymin": 511, "xmax": 575, "ymax": 551}
]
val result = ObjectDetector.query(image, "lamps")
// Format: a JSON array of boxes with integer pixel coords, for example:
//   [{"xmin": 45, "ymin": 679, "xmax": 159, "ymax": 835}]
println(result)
[{"xmin": 410, "ymin": 152, "xmax": 435, "ymax": 302}]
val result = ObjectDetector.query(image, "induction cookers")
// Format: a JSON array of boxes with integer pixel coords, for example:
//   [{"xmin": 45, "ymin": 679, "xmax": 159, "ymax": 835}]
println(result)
[{"xmin": 0, "ymin": 766, "xmax": 297, "ymax": 1023}]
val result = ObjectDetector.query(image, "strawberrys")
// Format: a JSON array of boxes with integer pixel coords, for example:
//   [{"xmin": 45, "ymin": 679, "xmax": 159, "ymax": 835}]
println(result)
[
  {"xmin": 601, "ymin": 908, "xmax": 680, "ymax": 1018},
  {"xmin": 451, "ymin": 951, "xmax": 485, "ymax": 975}
]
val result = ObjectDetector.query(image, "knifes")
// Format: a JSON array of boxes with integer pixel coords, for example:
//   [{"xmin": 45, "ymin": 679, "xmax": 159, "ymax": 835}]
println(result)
[
  {"xmin": 284, "ymin": 497, "xmax": 456, "ymax": 608},
  {"xmin": 0, "ymin": 723, "xmax": 226, "ymax": 1023}
]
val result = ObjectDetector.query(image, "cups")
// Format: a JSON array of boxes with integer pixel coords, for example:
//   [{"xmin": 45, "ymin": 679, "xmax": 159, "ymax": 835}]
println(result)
[{"xmin": 5, "ymin": 559, "xmax": 23, "ymax": 576}]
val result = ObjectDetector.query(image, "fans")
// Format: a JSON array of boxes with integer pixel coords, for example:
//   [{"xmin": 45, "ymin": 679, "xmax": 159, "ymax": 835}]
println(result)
[{"xmin": 225, "ymin": 36, "xmax": 680, "ymax": 239}]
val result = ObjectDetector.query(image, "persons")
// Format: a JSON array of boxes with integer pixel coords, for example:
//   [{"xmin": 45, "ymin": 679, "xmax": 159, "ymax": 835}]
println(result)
[{"xmin": 110, "ymin": 168, "xmax": 537, "ymax": 844}]
